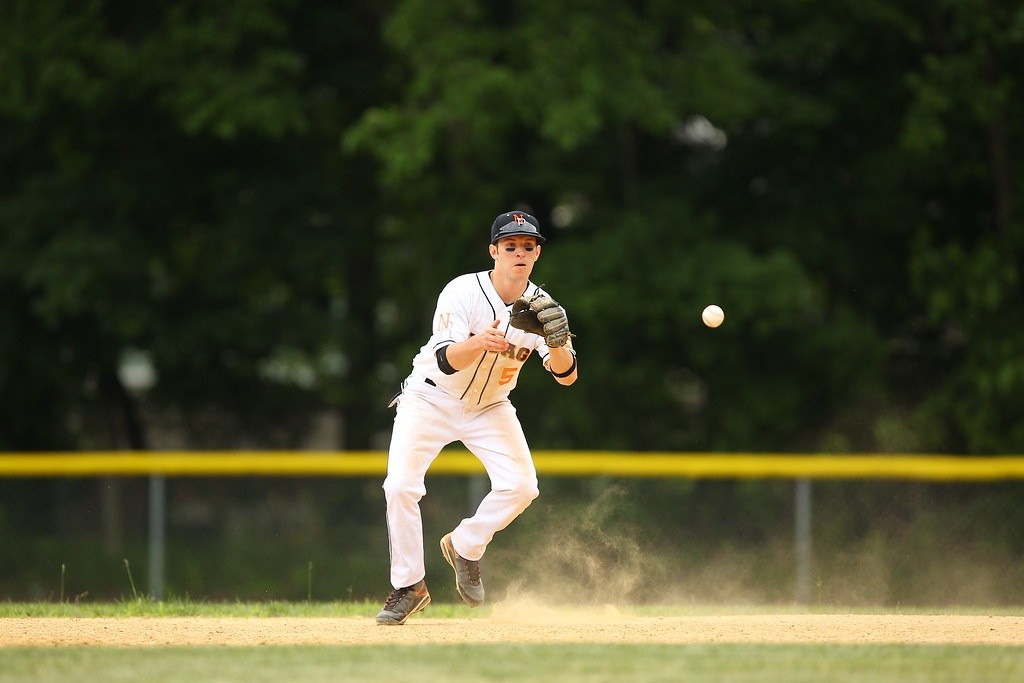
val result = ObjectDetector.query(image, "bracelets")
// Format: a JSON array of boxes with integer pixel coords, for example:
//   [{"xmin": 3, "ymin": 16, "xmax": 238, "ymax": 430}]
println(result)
[{"xmin": 550, "ymin": 348, "xmax": 577, "ymax": 378}]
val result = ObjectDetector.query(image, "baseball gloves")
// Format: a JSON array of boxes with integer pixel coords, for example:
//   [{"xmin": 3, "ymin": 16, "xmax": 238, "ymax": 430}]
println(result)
[{"xmin": 507, "ymin": 282, "xmax": 578, "ymax": 350}]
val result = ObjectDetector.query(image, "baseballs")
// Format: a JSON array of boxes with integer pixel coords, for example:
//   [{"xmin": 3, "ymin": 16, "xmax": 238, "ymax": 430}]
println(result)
[{"xmin": 701, "ymin": 303, "xmax": 727, "ymax": 328}]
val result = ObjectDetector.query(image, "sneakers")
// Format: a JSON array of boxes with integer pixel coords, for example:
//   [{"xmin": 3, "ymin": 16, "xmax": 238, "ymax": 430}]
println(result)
[
  {"xmin": 376, "ymin": 580, "xmax": 431, "ymax": 624},
  {"xmin": 440, "ymin": 533, "xmax": 485, "ymax": 608}
]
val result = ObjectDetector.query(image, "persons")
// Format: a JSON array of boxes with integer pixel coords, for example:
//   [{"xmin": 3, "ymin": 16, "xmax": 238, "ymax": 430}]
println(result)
[{"xmin": 374, "ymin": 210, "xmax": 578, "ymax": 627}]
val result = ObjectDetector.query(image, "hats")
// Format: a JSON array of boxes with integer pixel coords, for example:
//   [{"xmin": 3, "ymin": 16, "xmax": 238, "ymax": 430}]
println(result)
[{"xmin": 491, "ymin": 211, "xmax": 546, "ymax": 244}]
